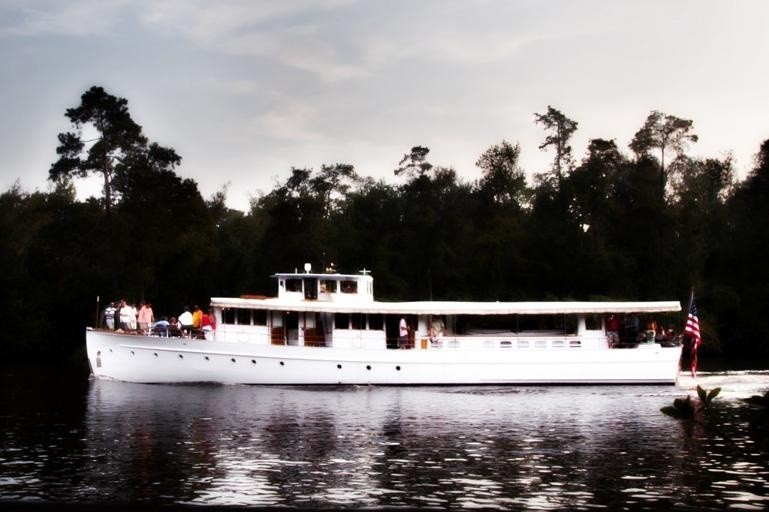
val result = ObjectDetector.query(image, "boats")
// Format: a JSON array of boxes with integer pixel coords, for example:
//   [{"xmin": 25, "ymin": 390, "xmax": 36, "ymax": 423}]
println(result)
[{"xmin": 86, "ymin": 259, "xmax": 704, "ymax": 387}]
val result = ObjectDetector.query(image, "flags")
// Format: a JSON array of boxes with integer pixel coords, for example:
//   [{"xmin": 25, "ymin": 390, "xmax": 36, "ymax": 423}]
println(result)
[{"xmin": 684, "ymin": 296, "xmax": 701, "ymax": 379}]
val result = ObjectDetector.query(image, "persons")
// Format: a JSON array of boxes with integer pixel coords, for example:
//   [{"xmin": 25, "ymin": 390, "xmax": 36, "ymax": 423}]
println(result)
[
  {"xmin": 398, "ymin": 314, "xmax": 411, "ymax": 350},
  {"xmin": 430, "ymin": 315, "xmax": 445, "ymax": 338},
  {"xmin": 625, "ymin": 314, "xmax": 676, "ymax": 347},
  {"xmin": 605, "ymin": 314, "xmax": 620, "ymax": 349},
  {"xmin": 101, "ymin": 295, "xmax": 215, "ymax": 342}
]
